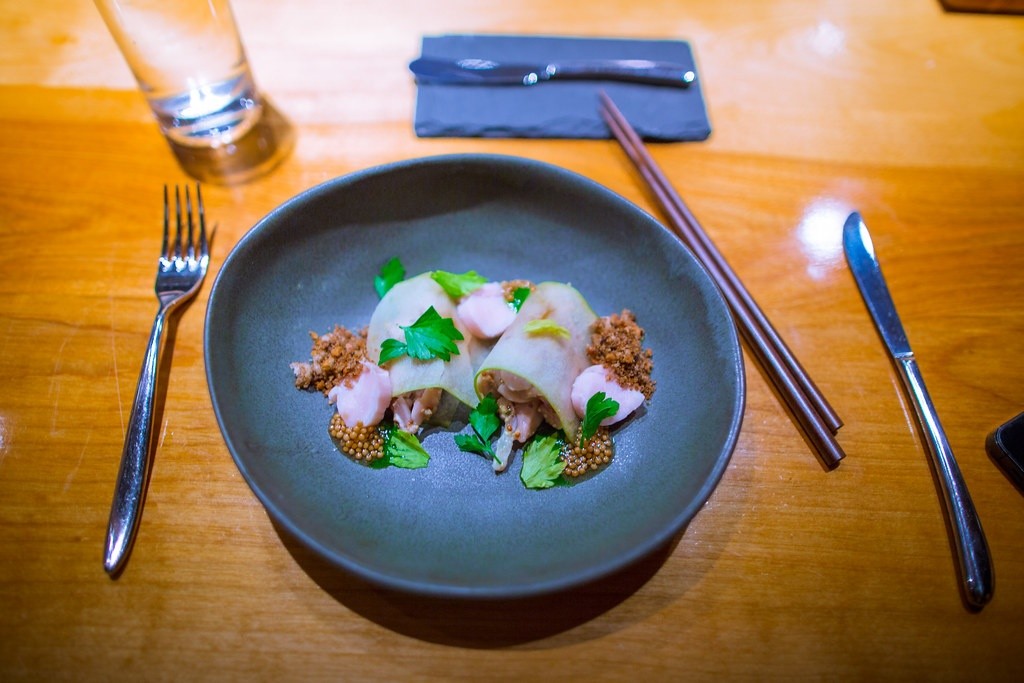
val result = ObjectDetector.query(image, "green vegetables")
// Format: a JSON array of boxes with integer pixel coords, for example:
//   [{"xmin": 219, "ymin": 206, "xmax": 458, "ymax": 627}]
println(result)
[{"xmin": 371, "ymin": 253, "xmax": 620, "ymax": 489}]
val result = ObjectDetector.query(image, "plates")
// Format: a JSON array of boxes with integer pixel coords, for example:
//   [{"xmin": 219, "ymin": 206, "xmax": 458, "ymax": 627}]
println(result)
[{"xmin": 203, "ymin": 151, "xmax": 748, "ymax": 597}]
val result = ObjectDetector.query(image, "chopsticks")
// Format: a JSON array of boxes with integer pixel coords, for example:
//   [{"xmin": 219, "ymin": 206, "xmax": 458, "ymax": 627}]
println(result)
[{"xmin": 598, "ymin": 89, "xmax": 847, "ymax": 471}]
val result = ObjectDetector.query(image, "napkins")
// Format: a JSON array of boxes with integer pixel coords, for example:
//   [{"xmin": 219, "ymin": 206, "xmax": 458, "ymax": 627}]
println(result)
[{"xmin": 415, "ymin": 34, "xmax": 710, "ymax": 143}]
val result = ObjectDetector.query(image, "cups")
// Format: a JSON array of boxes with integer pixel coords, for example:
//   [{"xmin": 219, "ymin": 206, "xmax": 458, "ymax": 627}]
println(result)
[{"xmin": 92, "ymin": 1, "xmax": 263, "ymax": 147}]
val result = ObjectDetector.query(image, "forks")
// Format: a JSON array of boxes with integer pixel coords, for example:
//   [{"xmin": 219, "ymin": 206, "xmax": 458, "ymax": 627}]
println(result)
[{"xmin": 102, "ymin": 183, "xmax": 210, "ymax": 573}]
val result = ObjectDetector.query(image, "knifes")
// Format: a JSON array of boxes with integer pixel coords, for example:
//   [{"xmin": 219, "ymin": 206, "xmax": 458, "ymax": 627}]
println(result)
[
  {"xmin": 842, "ymin": 212, "xmax": 996, "ymax": 610},
  {"xmin": 408, "ymin": 56, "xmax": 695, "ymax": 87}
]
